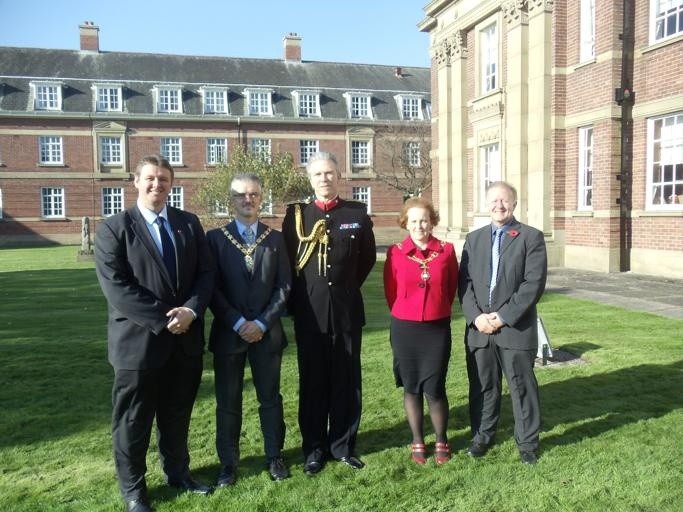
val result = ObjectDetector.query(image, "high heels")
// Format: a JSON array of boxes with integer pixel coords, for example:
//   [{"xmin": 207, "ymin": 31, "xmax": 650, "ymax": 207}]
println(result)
[
  {"xmin": 435, "ymin": 443, "xmax": 452, "ymax": 464},
  {"xmin": 411, "ymin": 442, "xmax": 426, "ymax": 463}
]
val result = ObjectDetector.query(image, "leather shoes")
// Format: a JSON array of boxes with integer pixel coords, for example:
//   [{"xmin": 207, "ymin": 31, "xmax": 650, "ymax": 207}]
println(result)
[
  {"xmin": 520, "ymin": 450, "xmax": 537, "ymax": 464},
  {"xmin": 469, "ymin": 443, "xmax": 495, "ymax": 456},
  {"xmin": 125, "ymin": 454, "xmax": 365, "ymax": 512}
]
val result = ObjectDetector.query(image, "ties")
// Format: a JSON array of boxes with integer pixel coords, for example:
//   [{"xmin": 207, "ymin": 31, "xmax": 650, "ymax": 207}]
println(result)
[
  {"xmin": 156, "ymin": 217, "xmax": 176, "ymax": 288},
  {"xmin": 244, "ymin": 228, "xmax": 256, "ymax": 247},
  {"xmin": 489, "ymin": 228, "xmax": 501, "ymax": 307}
]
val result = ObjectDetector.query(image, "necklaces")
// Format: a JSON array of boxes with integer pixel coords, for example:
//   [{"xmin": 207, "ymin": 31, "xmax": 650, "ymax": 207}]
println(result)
[
  {"xmin": 219, "ymin": 226, "xmax": 273, "ymax": 269},
  {"xmin": 397, "ymin": 242, "xmax": 447, "ymax": 282}
]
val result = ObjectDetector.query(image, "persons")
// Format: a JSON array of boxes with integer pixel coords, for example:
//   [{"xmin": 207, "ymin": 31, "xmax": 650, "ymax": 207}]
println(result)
[
  {"xmin": 456, "ymin": 181, "xmax": 547, "ymax": 465},
  {"xmin": 281, "ymin": 151, "xmax": 377, "ymax": 476},
  {"xmin": 95, "ymin": 155, "xmax": 212, "ymax": 512},
  {"xmin": 201, "ymin": 174, "xmax": 293, "ymax": 488},
  {"xmin": 383, "ymin": 199, "xmax": 458, "ymax": 465}
]
